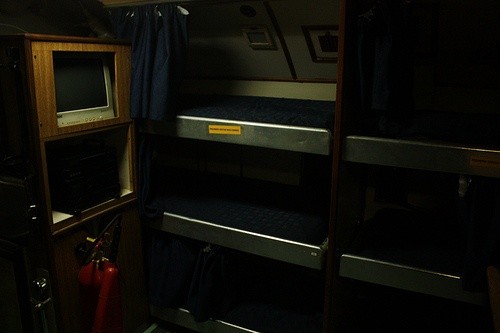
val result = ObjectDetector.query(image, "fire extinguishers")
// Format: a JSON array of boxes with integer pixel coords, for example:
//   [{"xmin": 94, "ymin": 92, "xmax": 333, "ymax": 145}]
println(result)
[{"xmin": 75, "ymin": 213, "xmax": 123, "ymax": 333}]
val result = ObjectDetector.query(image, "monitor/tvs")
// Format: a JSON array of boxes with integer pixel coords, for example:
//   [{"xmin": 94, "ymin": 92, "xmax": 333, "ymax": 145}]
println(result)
[{"xmin": 53, "ymin": 56, "xmax": 115, "ymax": 128}]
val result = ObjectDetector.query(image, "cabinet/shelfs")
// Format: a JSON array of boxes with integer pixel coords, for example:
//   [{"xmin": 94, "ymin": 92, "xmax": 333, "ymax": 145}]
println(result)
[
  {"xmin": 0, "ymin": 32, "xmax": 148, "ymax": 333},
  {"xmin": 142, "ymin": 0, "xmax": 500, "ymax": 333}
]
